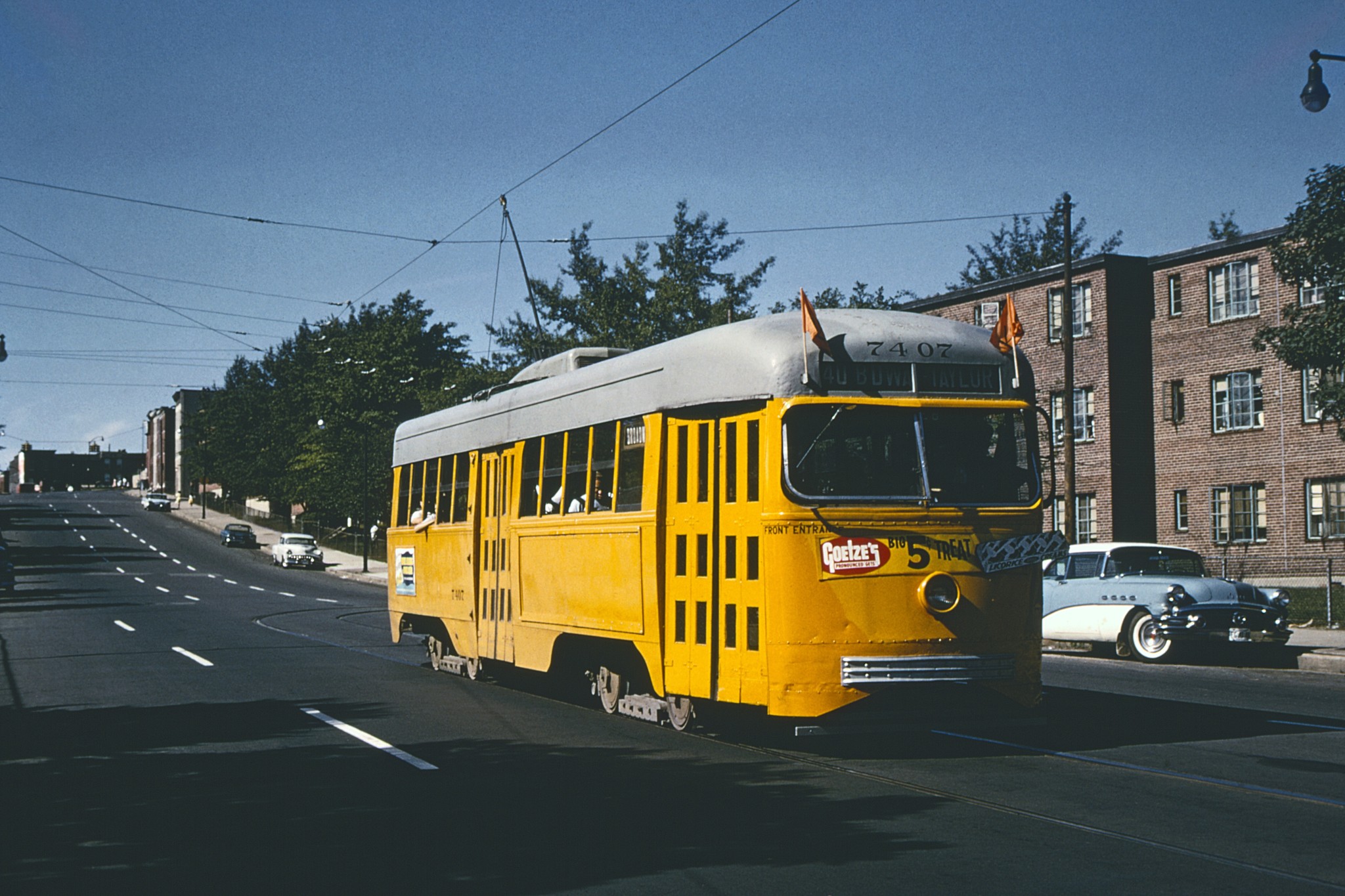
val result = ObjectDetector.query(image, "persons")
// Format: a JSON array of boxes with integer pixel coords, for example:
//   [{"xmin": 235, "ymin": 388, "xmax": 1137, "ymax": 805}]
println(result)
[
  {"xmin": 112, "ymin": 477, "xmax": 130, "ymax": 489},
  {"xmin": 414, "ymin": 503, "xmax": 437, "ymax": 532},
  {"xmin": 175, "ymin": 491, "xmax": 181, "ymax": 509},
  {"xmin": 533, "ymin": 484, "xmax": 562, "ymax": 515},
  {"xmin": 188, "ymin": 492, "xmax": 193, "ymax": 507},
  {"xmin": 411, "ymin": 500, "xmax": 422, "ymax": 524},
  {"xmin": 567, "ymin": 470, "xmax": 611, "ymax": 513},
  {"xmin": 370, "ymin": 523, "xmax": 379, "ymax": 550}
]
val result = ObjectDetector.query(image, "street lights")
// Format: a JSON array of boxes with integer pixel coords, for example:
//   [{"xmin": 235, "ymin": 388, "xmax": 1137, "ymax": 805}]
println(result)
[
  {"xmin": 88, "ymin": 436, "xmax": 104, "ymax": 489},
  {"xmin": 319, "ymin": 419, "xmax": 369, "ymax": 572},
  {"xmin": 177, "ymin": 425, "xmax": 207, "ymax": 519}
]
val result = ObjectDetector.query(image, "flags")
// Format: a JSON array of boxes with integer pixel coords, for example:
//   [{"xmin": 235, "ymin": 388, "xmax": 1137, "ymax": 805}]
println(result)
[
  {"xmin": 989, "ymin": 296, "xmax": 1024, "ymax": 354},
  {"xmin": 801, "ymin": 290, "xmax": 828, "ymax": 350}
]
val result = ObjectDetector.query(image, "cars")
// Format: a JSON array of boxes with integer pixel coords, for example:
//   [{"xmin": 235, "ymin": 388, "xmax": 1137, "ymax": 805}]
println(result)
[
  {"xmin": 219, "ymin": 523, "xmax": 256, "ymax": 548},
  {"xmin": 1041, "ymin": 542, "xmax": 1295, "ymax": 663},
  {"xmin": 142, "ymin": 492, "xmax": 171, "ymax": 512},
  {"xmin": 272, "ymin": 533, "xmax": 323, "ymax": 570}
]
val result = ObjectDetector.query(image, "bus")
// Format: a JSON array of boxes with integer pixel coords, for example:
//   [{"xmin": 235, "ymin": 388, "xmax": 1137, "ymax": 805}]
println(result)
[{"xmin": 388, "ymin": 195, "xmax": 1056, "ymax": 744}]
